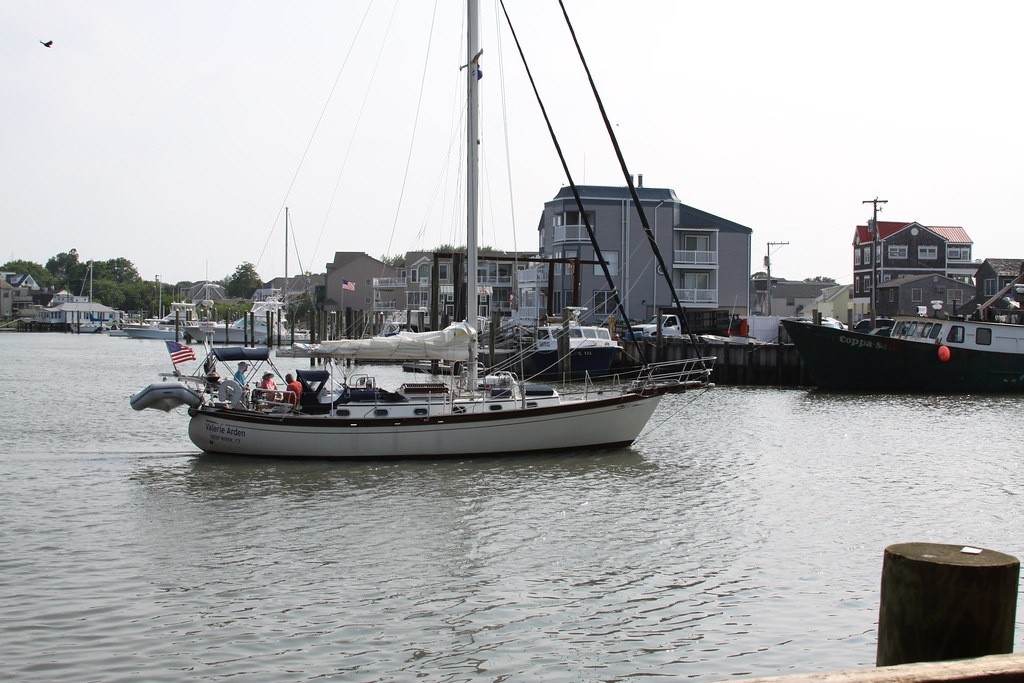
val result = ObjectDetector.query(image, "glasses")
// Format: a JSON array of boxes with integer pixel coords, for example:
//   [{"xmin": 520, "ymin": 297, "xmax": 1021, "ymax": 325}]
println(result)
[{"xmin": 266, "ymin": 374, "xmax": 271, "ymax": 376}]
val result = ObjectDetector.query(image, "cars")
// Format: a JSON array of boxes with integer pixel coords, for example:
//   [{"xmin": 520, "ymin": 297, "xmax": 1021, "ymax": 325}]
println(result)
[{"xmin": 808, "ymin": 315, "xmax": 896, "ymax": 335}]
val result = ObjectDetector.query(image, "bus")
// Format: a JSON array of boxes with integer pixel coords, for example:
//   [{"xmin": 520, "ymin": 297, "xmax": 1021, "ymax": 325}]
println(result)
[{"xmin": 536, "ymin": 325, "xmax": 618, "ymax": 351}]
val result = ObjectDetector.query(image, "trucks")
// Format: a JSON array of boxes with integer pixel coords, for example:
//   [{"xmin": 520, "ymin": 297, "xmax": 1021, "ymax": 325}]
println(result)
[{"xmin": 627, "ymin": 305, "xmax": 732, "ymax": 339}]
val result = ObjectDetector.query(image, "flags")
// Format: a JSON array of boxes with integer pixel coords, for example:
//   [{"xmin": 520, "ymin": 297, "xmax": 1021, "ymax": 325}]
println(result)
[
  {"xmin": 342, "ymin": 279, "xmax": 356, "ymax": 292},
  {"xmin": 165, "ymin": 340, "xmax": 196, "ymax": 364}
]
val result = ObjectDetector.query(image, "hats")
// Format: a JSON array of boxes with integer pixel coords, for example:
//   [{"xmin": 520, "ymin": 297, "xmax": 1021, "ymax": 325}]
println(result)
[
  {"xmin": 262, "ymin": 371, "xmax": 275, "ymax": 379},
  {"xmin": 237, "ymin": 362, "xmax": 251, "ymax": 372}
]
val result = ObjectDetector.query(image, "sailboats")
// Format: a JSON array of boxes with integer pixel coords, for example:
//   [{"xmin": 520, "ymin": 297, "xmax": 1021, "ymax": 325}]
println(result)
[
  {"xmin": 130, "ymin": 0, "xmax": 716, "ymax": 453},
  {"xmin": 69, "ymin": 258, "xmax": 101, "ymax": 333}
]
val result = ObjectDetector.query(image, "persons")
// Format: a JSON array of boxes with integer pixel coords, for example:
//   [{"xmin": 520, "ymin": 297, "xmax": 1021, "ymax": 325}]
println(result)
[
  {"xmin": 283, "ymin": 374, "xmax": 303, "ymax": 405},
  {"xmin": 234, "ymin": 361, "xmax": 250, "ymax": 390},
  {"xmin": 261, "ymin": 370, "xmax": 281, "ymax": 401}
]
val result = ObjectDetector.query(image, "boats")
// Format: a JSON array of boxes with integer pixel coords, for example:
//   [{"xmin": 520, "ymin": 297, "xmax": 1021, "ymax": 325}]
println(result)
[
  {"xmin": 180, "ymin": 296, "xmax": 316, "ymax": 345},
  {"xmin": 778, "ymin": 273, "xmax": 1024, "ymax": 396},
  {"xmin": 109, "ymin": 325, "xmax": 130, "ymax": 336},
  {"xmin": 123, "ymin": 317, "xmax": 189, "ymax": 340}
]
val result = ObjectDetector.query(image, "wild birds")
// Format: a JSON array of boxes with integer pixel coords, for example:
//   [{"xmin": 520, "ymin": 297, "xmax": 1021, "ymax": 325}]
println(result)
[{"xmin": 40, "ymin": 40, "xmax": 53, "ymax": 48}]
